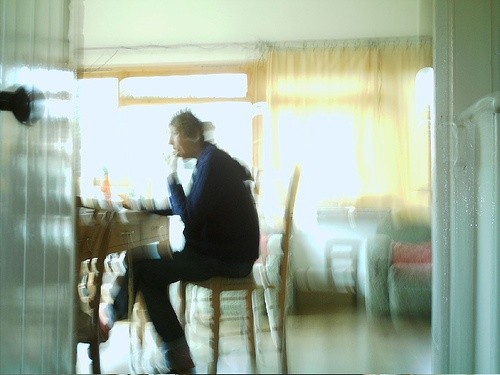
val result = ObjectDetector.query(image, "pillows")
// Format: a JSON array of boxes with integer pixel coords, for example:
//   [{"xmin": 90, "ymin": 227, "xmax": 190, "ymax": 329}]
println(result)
[{"xmin": 391, "ymin": 237, "xmax": 435, "ymax": 264}]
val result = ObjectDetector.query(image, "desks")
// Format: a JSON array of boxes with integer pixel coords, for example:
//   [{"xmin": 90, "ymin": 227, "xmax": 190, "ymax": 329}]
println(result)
[{"xmin": 79, "ymin": 211, "xmax": 180, "ymax": 374}]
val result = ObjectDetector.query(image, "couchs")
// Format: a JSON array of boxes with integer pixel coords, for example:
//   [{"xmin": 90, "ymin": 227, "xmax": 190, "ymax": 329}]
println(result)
[{"xmin": 359, "ymin": 215, "xmax": 433, "ymax": 318}]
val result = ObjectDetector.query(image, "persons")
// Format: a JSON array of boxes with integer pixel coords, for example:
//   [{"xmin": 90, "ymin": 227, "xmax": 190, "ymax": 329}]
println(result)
[{"xmin": 99, "ymin": 111, "xmax": 260, "ymax": 375}]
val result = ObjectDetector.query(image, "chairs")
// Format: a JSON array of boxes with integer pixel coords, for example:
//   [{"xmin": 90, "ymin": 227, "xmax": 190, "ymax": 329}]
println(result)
[
  {"xmin": 170, "ymin": 230, "xmax": 295, "ymax": 335},
  {"xmin": 177, "ymin": 162, "xmax": 301, "ymax": 374}
]
